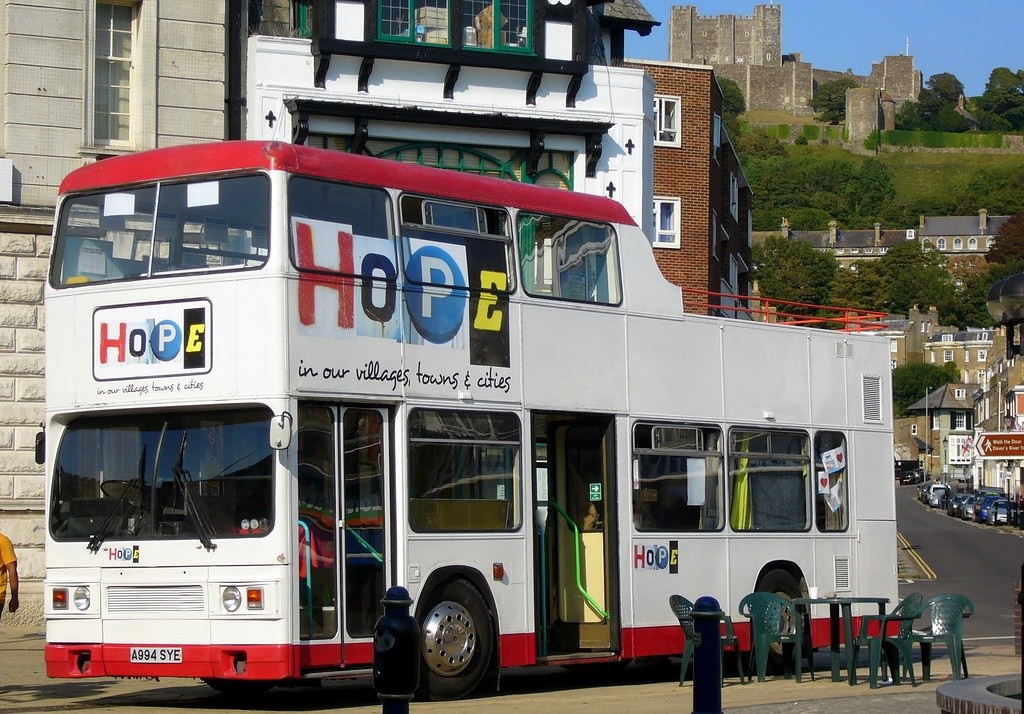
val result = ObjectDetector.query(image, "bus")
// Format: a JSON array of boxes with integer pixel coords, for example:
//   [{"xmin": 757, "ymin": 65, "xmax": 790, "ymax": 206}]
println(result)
[{"xmin": 43, "ymin": 140, "xmax": 899, "ymax": 704}]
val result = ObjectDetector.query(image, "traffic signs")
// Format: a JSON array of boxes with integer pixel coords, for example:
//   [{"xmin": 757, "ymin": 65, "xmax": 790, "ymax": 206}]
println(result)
[{"xmin": 975, "ymin": 432, "xmax": 1024, "ymax": 460}]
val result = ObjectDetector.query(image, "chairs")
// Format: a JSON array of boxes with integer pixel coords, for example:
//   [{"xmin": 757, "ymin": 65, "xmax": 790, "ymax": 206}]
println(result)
[
  {"xmin": 849, "ymin": 592, "xmax": 922, "ymax": 688},
  {"xmin": 739, "ymin": 592, "xmax": 805, "ymax": 684},
  {"xmin": 669, "ymin": 595, "xmax": 745, "ymax": 687},
  {"xmin": 903, "ymin": 593, "xmax": 975, "ymax": 680}
]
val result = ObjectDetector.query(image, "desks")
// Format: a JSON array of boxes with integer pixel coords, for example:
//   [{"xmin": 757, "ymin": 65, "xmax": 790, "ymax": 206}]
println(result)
[{"xmin": 790, "ymin": 597, "xmax": 891, "ymax": 687}]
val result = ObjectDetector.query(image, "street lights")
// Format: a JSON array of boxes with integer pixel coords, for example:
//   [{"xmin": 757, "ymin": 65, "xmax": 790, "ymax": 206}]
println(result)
[{"xmin": 926, "ymin": 387, "xmax": 933, "ymax": 474}]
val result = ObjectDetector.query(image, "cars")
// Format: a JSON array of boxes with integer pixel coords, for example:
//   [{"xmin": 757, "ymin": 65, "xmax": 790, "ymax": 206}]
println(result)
[{"xmin": 900, "ymin": 468, "xmax": 1021, "ymax": 526}]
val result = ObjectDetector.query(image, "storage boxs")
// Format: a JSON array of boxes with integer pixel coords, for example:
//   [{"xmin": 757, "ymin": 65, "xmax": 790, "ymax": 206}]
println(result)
[
  {"xmin": 414, "ymin": 6, "xmax": 447, "ymax": 28},
  {"xmin": 422, "ymin": 27, "xmax": 448, "ymax": 44},
  {"xmin": 473, "ymin": 5, "xmax": 508, "ymax": 30},
  {"xmin": 477, "ymin": 30, "xmax": 506, "ymax": 48}
]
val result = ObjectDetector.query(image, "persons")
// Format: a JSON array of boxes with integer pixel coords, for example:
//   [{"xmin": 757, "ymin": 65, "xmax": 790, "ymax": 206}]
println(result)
[
  {"xmin": 584, "ymin": 501, "xmax": 603, "ymax": 529},
  {"xmin": 0, "ymin": 532, "xmax": 19, "ymax": 619}
]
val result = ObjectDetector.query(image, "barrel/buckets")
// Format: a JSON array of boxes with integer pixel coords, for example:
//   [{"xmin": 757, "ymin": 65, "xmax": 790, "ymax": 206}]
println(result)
[{"xmin": 322, "ymin": 607, "xmax": 337, "ymax": 639}]
